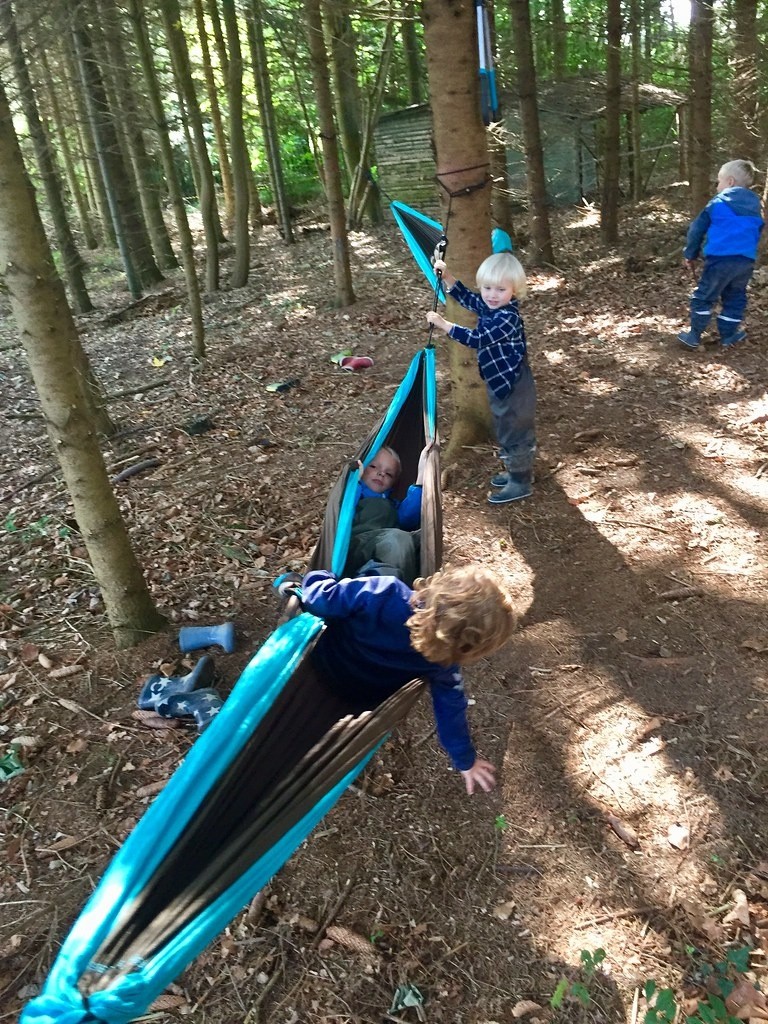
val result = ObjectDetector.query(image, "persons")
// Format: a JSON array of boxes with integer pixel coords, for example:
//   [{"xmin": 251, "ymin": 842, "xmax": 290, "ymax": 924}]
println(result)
[
  {"xmin": 676, "ymin": 159, "xmax": 766, "ymax": 349},
  {"xmin": 426, "ymin": 253, "xmax": 537, "ymax": 504},
  {"xmin": 301, "ymin": 564, "xmax": 516, "ymax": 797},
  {"xmin": 348, "ymin": 442, "xmax": 433, "ymax": 581}
]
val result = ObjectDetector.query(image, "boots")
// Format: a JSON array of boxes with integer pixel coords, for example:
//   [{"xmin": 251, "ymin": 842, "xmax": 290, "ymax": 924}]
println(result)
[
  {"xmin": 340, "ymin": 356, "xmax": 374, "ymax": 372},
  {"xmin": 491, "ymin": 473, "xmax": 535, "ymax": 487},
  {"xmin": 677, "ymin": 311, "xmax": 711, "ymax": 347},
  {"xmin": 0, "ymin": 743, "xmax": 25, "ymax": 782},
  {"xmin": 488, "ymin": 463, "xmax": 533, "ymax": 503},
  {"xmin": 154, "ymin": 687, "xmax": 224, "ymax": 734},
  {"xmin": 717, "ymin": 315, "xmax": 747, "ymax": 347},
  {"xmin": 179, "ymin": 622, "xmax": 235, "ymax": 654},
  {"xmin": 138, "ymin": 656, "xmax": 215, "ymax": 709}
]
